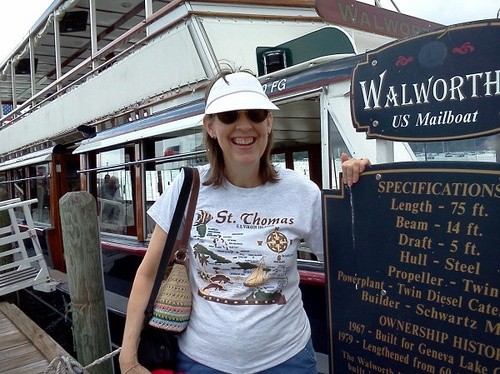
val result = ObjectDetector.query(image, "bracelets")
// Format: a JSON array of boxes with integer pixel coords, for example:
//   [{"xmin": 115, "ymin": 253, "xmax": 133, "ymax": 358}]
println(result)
[{"xmin": 121, "ymin": 363, "xmax": 141, "ymax": 374}]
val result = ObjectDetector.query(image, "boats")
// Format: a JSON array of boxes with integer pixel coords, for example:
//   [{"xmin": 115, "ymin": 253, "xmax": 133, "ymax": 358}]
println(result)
[{"xmin": 0, "ymin": 0, "xmax": 499, "ymax": 355}]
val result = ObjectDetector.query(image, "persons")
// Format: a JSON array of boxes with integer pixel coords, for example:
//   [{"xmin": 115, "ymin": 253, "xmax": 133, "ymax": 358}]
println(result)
[
  {"xmin": 16, "ymin": 164, "xmax": 125, "ymax": 231},
  {"xmin": 117, "ymin": 69, "xmax": 373, "ymax": 374}
]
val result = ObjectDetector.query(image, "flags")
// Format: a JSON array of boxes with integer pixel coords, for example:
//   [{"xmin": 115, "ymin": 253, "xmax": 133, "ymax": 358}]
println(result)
[
  {"xmin": 163, "ymin": 144, "xmax": 181, "ymax": 159},
  {"xmin": 3, "ymin": 104, "xmax": 18, "ymax": 127}
]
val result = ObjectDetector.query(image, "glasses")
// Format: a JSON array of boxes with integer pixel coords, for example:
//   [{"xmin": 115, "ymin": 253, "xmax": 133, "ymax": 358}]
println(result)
[{"xmin": 213, "ymin": 109, "xmax": 270, "ymax": 125}]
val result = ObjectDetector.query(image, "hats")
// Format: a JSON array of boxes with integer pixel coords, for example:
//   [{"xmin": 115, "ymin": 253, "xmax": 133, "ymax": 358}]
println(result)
[{"xmin": 204, "ymin": 72, "xmax": 280, "ymax": 115}]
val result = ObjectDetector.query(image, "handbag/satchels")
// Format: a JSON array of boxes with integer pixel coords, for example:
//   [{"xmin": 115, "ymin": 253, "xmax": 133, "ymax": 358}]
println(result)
[
  {"xmin": 148, "ymin": 168, "xmax": 201, "ymax": 332},
  {"xmin": 137, "ymin": 313, "xmax": 180, "ymax": 373}
]
